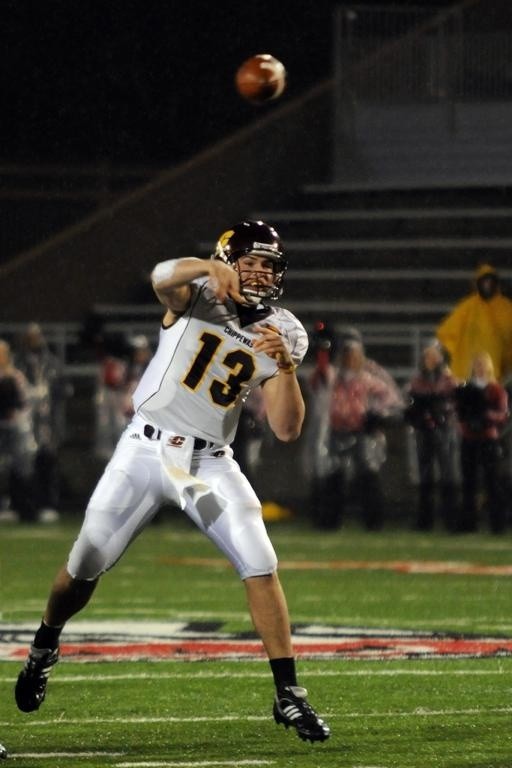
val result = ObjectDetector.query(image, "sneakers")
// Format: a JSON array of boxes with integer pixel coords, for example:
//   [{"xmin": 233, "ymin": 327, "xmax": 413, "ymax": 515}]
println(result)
[
  {"xmin": 15, "ymin": 642, "xmax": 59, "ymax": 712},
  {"xmin": 273, "ymin": 686, "xmax": 331, "ymax": 744}
]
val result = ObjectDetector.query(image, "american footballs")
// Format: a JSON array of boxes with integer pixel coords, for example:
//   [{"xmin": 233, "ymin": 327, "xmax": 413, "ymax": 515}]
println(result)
[{"xmin": 236, "ymin": 54, "xmax": 286, "ymax": 102}]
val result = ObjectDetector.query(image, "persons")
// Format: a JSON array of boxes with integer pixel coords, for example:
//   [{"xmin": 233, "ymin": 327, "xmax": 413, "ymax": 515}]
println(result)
[
  {"xmin": 235, "ymin": 263, "xmax": 511, "ymax": 536},
  {"xmin": 14, "ymin": 222, "xmax": 333, "ymax": 745},
  {"xmin": 1, "ymin": 312, "xmax": 154, "ymax": 525}
]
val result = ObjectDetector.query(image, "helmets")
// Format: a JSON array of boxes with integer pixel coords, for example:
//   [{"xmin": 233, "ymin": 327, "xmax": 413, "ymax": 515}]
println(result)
[{"xmin": 210, "ymin": 219, "xmax": 290, "ymax": 308}]
